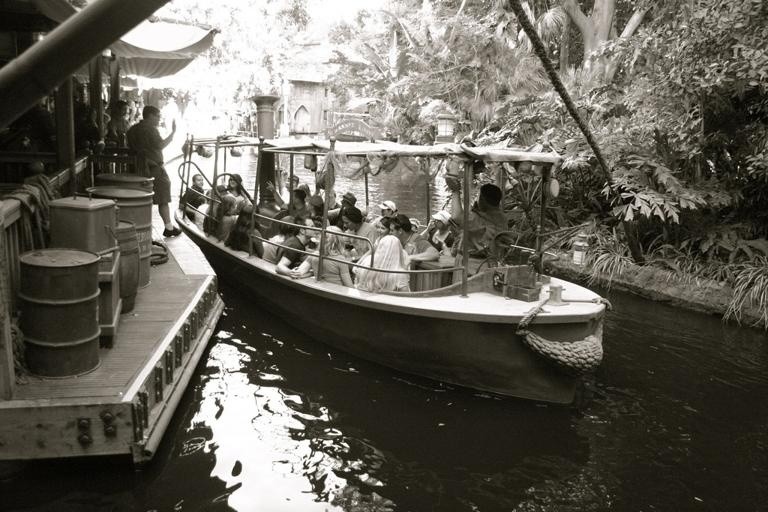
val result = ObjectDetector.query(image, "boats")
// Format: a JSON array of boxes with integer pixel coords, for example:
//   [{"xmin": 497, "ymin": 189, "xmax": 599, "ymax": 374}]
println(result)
[{"xmin": 174, "ymin": 135, "xmax": 612, "ymax": 403}]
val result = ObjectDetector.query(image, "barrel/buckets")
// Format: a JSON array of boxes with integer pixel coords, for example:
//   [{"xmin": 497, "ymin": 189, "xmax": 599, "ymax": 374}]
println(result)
[
  {"xmin": 94, "ymin": 171, "xmax": 155, "ymax": 190},
  {"xmin": 572, "ymin": 234, "xmax": 588, "ymax": 265},
  {"xmin": 114, "ymin": 218, "xmax": 140, "ymax": 314},
  {"xmin": 85, "ymin": 182, "xmax": 154, "ymax": 289},
  {"xmin": 16, "ymin": 247, "xmax": 102, "ymax": 377}
]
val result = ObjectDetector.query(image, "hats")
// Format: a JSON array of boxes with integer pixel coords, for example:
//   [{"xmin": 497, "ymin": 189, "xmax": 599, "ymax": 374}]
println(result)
[
  {"xmin": 341, "ymin": 192, "xmax": 357, "ymax": 208},
  {"xmin": 304, "ymin": 194, "xmax": 324, "ymax": 207},
  {"xmin": 376, "ymin": 199, "xmax": 396, "ymax": 213},
  {"xmin": 388, "ymin": 214, "xmax": 420, "ymax": 232},
  {"xmin": 431, "ymin": 209, "xmax": 451, "ymax": 226},
  {"xmin": 479, "ymin": 183, "xmax": 503, "ymax": 208}
]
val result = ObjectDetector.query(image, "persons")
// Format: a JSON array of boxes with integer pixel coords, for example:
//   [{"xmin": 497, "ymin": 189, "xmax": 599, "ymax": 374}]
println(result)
[
  {"xmin": 443, "ymin": 170, "xmax": 508, "ymax": 283},
  {"xmin": 125, "ymin": 105, "xmax": 183, "ymax": 237},
  {"xmin": 179, "ymin": 172, "xmax": 479, "ymax": 293},
  {"xmin": 1, "ymin": 93, "xmax": 140, "ymax": 173}
]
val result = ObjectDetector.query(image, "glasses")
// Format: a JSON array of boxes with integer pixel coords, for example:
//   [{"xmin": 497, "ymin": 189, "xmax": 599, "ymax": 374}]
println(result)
[{"xmin": 151, "ymin": 114, "xmax": 161, "ymax": 118}]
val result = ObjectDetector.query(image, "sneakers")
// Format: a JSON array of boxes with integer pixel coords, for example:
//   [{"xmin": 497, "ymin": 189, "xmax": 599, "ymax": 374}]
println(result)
[{"xmin": 162, "ymin": 225, "xmax": 183, "ymax": 237}]
